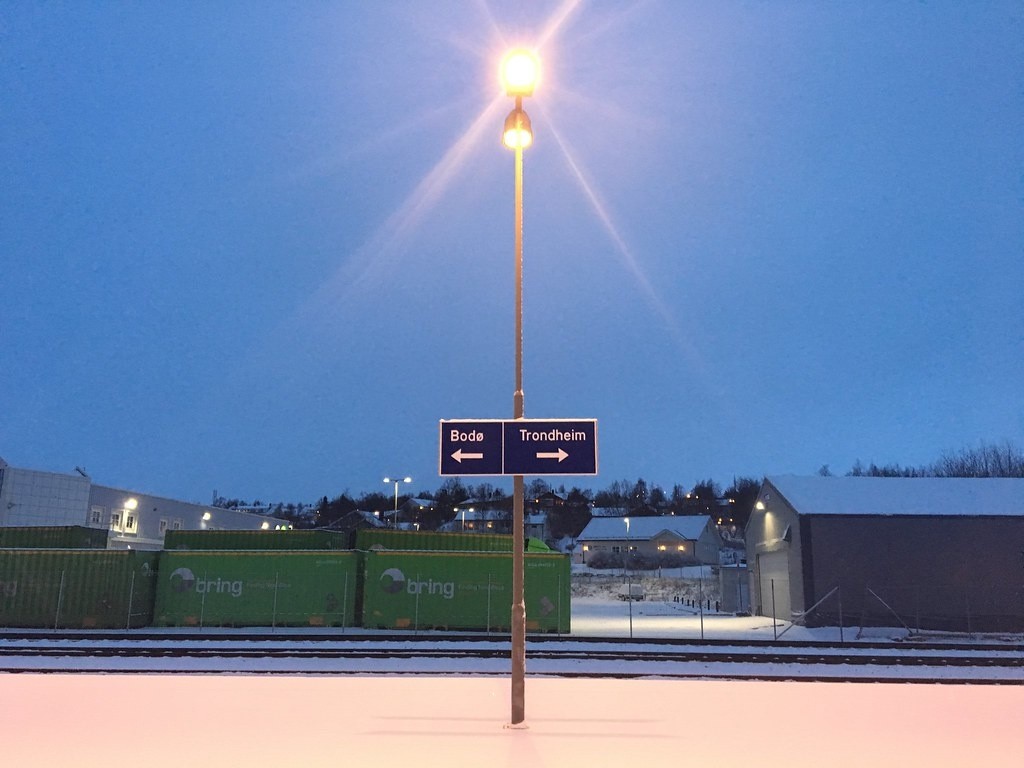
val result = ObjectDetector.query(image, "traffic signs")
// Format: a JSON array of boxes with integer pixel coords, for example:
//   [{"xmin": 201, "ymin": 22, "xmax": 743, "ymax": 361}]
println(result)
[{"xmin": 438, "ymin": 419, "xmax": 597, "ymax": 476}]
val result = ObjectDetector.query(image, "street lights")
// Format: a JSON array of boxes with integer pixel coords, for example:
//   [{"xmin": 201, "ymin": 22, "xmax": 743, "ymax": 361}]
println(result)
[
  {"xmin": 582, "ymin": 545, "xmax": 588, "ymax": 563},
  {"xmin": 624, "ymin": 518, "xmax": 630, "ymax": 555},
  {"xmin": 497, "ymin": 46, "xmax": 547, "ymax": 724},
  {"xmin": 454, "ymin": 508, "xmax": 475, "ymax": 533},
  {"xmin": 384, "ymin": 478, "xmax": 412, "ymax": 529}
]
